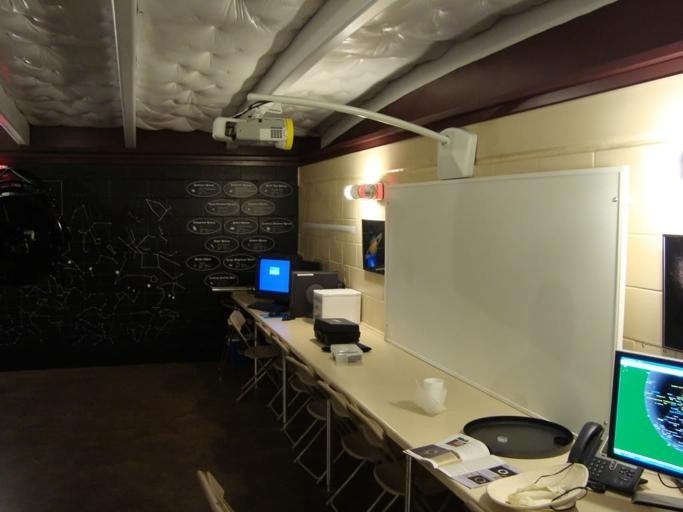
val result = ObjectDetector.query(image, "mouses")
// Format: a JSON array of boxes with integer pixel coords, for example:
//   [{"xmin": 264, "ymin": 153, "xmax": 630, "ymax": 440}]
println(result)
[{"xmin": 268, "ymin": 311, "xmax": 280, "ymax": 317}]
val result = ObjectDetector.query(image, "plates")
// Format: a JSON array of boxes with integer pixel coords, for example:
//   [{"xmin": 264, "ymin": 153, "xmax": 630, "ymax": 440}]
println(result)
[
  {"xmin": 487, "ymin": 462, "xmax": 589, "ymax": 511},
  {"xmin": 463, "ymin": 416, "xmax": 576, "ymax": 460}
]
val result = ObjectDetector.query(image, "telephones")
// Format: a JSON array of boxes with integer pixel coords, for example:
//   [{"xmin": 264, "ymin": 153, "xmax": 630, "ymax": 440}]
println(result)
[{"xmin": 568, "ymin": 422, "xmax": 645, "ymax": 496}]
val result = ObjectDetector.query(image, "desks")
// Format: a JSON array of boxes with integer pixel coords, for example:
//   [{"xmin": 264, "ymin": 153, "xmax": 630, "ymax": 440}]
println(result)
[{"xmin": 230, "ymin": 284, "xmax": 683, "ymax": 512}]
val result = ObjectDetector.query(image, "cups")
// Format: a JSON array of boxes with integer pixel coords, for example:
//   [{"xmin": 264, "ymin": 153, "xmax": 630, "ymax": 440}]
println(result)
[
  {"xmin": 422, "ymin": 378, "xmax": 444, "ymax": 414},
  {"xmin": 336, "ymin": 350, "xmax": 347, "ymax": 368}
]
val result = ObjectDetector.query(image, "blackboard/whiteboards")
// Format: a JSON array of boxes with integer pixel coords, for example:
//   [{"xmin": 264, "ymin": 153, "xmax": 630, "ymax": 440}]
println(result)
[{"xmin": 383, "ymin": 167, "xmax": 628, "ymax": 438}]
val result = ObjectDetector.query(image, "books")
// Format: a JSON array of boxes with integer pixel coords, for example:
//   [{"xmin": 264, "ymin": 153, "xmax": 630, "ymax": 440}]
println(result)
[{"xmin": 401, "ymin": 432, "xmax": 522, "ymax": 489}]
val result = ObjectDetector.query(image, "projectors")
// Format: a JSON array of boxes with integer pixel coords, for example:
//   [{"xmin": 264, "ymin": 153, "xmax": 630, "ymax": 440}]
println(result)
[{"xmin": 212, "ymin": 101, "xmax": 293, "ymax": 149}]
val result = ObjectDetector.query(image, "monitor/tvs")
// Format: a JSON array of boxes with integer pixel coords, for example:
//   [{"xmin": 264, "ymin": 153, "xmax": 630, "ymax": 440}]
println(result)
[
  {"xmin": 255, "ymin": 257, "xmax": 291, "ymax": 304},
  {"xmin": 608, "ymin": 348, "xmax": 683, "ymax": 512}
]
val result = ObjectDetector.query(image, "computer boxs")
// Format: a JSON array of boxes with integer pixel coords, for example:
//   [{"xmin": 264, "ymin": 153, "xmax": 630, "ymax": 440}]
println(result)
[{"xmin": 288, "ymin": 271, "xmax": 339, "ymax": 319}]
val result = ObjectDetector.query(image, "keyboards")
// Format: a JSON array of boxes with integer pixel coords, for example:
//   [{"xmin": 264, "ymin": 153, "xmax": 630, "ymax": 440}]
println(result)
[{"xmin": 248, "ymin": 301, "xmax": 288, "ymax": 313}]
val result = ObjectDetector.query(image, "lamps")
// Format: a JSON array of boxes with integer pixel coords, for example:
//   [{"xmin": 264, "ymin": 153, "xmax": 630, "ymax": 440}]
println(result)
[{"xmin": 342, "ymin": 183, "xmax": 385, "ymax": 200}]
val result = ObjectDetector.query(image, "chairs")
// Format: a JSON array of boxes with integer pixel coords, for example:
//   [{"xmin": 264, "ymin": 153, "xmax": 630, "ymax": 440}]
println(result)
[
  {"xmin": 228, "ymin": 309, "xmax": 284, "ymax": 407},
  {"xmin": 255, "ymin": 323, "xmax": 305, "ymax": 420},
  {"xmin": 196, "ymin": 469, "xmax": 234, "ymax": 511},
  {"xmin": 325, "ymin": 401, "xmax": 450, "ymax": 512},
  {"xmin": 292, "ymin": 378, "xmax": 379, "ymax": 483},
  {"xmin": 269, "ymin": 355, "xmax": 329, "ymax": 430},
  {"xmin": 267, "ymin": 335, "xmax": 309, "ymax": 420}
]
